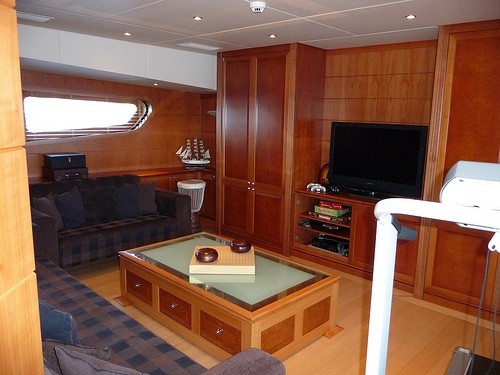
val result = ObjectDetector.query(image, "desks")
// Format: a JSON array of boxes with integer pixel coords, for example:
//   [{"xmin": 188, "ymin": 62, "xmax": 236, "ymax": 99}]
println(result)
[{"xmin": 177, "ymin": 180, "xmax": 206, "ymax": 233}]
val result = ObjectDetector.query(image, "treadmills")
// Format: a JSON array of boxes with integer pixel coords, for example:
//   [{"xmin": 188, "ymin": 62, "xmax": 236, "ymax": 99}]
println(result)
[{"xmin": 364, "ymin": 161, "xmax": 500, "ymax": 375}]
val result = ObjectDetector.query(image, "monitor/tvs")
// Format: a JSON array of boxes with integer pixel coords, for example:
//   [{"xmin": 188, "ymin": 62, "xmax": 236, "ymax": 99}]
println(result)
[{"xmin": 328, "ymin": 121, "xmax": 430, "ymax": 200}]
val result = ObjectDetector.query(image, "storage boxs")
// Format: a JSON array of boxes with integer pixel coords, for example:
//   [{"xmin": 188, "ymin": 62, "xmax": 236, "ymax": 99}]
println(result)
[{"xmin": 314, "ymin": 205, "xmax": 350, "ymax": 217}]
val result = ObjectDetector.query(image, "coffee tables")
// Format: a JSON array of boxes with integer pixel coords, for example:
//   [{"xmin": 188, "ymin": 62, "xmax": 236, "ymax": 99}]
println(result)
[{"xmin": 119, "ymin": 232, "xmax": 342, "ymax": 364}]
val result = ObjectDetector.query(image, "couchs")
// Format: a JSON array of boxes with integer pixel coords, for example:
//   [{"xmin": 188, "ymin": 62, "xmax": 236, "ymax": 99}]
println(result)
[
  {"xmin": 29, "ymin": 173, "xmax": 192, "ymax": 270},
  {"xmin": 35, "ymin": 255, "xmax": 286, "ymax": 375}
]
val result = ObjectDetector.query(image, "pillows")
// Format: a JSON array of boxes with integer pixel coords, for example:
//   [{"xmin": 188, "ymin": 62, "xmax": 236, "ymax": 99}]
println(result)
[
  {"xmin": 32, "ymin": 193, "xmax": 65, "ymax": 230},
  {"xmin": 41, "ymin": 341, "xmax": 111, "ymax": 373},
  {"xmin": 54, "ymin": 187, "xmax": 88, "ymax": 229},
  {"xmin": 55, "ymin": 344, "xmax": 150, "ymax": 375},
  {"xmin": 140, "ymin": 182, "xmax": 157, "ymax": 215},
  {"xmin": 38, "ymin": 297, "xmax": 80, "ymax": 345},
  {"xmin": 94, "ymin": 184, "xmax": 138, "ymax": 220}
]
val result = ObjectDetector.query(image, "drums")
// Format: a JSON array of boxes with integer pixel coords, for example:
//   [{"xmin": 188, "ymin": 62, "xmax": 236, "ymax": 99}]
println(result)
[{"xmin": 177, "ymin": 180, "xmax": 206, "ymax": 232}]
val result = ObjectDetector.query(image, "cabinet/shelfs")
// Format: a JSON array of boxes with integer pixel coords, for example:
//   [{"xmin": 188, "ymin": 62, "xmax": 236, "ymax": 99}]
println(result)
[
  {"xmin": 295, "ymin": 188, "xmax": 420, "ymax": 289},
  {"xmin": 415, "ymin": 20, "xmax": 500, "ymax": 323},
  {"xmin": 216, "ymin": 42, "xmax": 326, "ymax": 256},
  {"xmin": 141, "ymin": 175, "xmax": 216, "ymax": 222}
]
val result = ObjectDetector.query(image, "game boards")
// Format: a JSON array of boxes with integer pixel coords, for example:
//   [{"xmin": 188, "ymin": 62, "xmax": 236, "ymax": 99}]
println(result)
[{"xmin": 189, "ymin": 246, "xmax": 256, "ymax": 275}]
